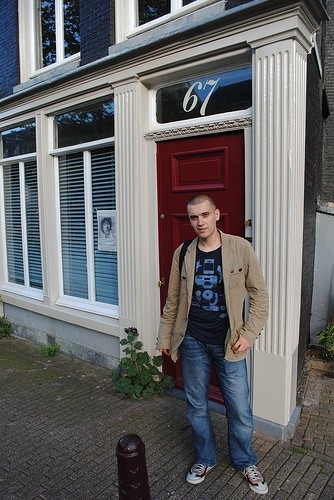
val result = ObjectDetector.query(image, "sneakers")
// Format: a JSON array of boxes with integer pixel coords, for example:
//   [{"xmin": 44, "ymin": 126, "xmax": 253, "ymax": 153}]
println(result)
[
  {"xmin": 240, "ymin": 464, "xmax": 269, "ymax": 494},
  {"xmin": 186, "ymin": 461, "xmax": 219, "ymax": 484}
]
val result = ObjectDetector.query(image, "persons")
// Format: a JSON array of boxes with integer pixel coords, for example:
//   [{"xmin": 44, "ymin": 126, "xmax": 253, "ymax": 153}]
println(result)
[
  {"xmin": 156, "ymin": 195, "xmax": 270, "ymax": 494},
  {"xmin": 101, "ymin": 217, "xmax": 114, "ymax": 240}
]
